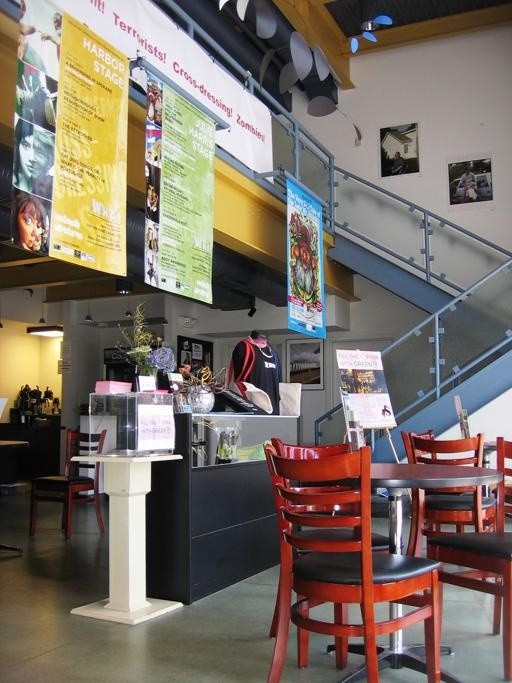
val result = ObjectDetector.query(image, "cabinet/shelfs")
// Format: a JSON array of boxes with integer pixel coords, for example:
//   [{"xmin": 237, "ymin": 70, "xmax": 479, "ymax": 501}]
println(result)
[{"xmin": 115, "ymin": 396, "xmax": 304, "ymax": 606}]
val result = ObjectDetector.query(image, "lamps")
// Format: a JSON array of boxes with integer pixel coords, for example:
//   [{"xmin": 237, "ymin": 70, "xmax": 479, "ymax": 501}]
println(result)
[
  {"xmin": 126, "ymin": 294, "xmax": 132, "ymax": 317},
  {"xmin": 27, "ymin": 326, "xmax": 63, "ymax": 338},
  {"xmin": 84, "ymin": 270, "xmax": 92, "ymax": 320},
  {"xmin": 248, "ymin": 300, "xmax": 256, "ymax": 316},
  {"xmin": 361, "ymin": 20, "xmax": 374, "ymax": 31},
  {"xmin": 341, "ymin": 36, "xmax": 359, "ymax": 55},
  {"xmin": 38, "ymin": 287, "xmax": 47, "ymax": 323},
  {"xmin": 116, "ymin": 272, "xmax": 134, "ymax": 295}
]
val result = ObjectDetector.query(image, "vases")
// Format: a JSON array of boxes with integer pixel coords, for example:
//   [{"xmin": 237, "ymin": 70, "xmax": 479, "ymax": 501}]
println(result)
[
  {"xmin": 188, "ymin": 386, "xmax": 215, "ymax": 413},
  {"xmin": 136, "ymin": 365, "xmax": 157, "ymax": 376}
]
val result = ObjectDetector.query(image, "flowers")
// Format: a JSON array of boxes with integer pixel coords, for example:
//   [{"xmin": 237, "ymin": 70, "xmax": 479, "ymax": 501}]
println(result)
[{"xmin": 112, "ymin": 300, "xmax": 178, "ymax": 376}]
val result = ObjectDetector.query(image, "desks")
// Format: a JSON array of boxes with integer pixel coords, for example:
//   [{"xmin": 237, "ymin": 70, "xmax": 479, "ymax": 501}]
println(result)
[
  {"xmin": 326, "ymin": 463, "xmax": 504, "ymax": 683},
  {"xmin": 70, "ymin": 454, "xmax": 185, "ymax": 625},
  {"xmin": 0, "ymin": 440, "xmax": 29, "ymax": 553},
  {"xmin": 11, "ymin": 415, "xmax": 60, "ymax": 479}
]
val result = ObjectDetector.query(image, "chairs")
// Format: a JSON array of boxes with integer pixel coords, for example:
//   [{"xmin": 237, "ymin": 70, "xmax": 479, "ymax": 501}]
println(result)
[
  {"xmin": 261, "ymin": 443, "xmax": 441, "ymax": 682},
  {"xmin": 402, "ymin": 431, "xmax": 496, "ymax": 567},
  {"xmin": 426, "ymin": 437, "xmax": 512, "ymax": 683},
  {"xmin": 271, "ymin": 436, "xmax": 404, "ymax": 636},
  {"xmin": 28, "ymin": 429, "xmax": 107, "ymax": 539}
]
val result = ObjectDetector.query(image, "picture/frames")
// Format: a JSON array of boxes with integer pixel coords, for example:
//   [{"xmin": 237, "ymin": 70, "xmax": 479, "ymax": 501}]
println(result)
[{"xmin": 286, "ymin": 339, "xmax": 324, "ymax": 390}]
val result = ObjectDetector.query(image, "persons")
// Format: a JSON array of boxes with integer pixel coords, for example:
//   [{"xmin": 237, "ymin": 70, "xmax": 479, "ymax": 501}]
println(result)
[
  {"xmin": 457, "ymin": 163, "xmax": 478, "ymax": 202},
  {"xmin": 182, "ymin": 352, "xmax": 192, "ymax": 366},
  {"xmin": 339, "ymin": 368, "xmax": 387, "ymax": 394},
  {"xmin": 140, "ymin": 78, "xmax": 163, "ymax": 286},
  {"xmin": 391, "ymin": 150, "xmax": 406, "ymax": 174},
  {"xmin": 10, "ymin": 0, "xmax": 66, "ymax": 258},
  {"xmin": 52, "ymin": 397, "xmax": 62, "ymax": 414},
  {"xmin": 231, "ymin": 327, "xmax": 280, "ymax": 418}
]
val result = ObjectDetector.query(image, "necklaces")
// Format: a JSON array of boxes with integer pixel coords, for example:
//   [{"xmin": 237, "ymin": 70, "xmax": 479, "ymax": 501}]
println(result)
[{"xmin": 248, "ymin": 335, "xmax": 273, "ymax": 358}]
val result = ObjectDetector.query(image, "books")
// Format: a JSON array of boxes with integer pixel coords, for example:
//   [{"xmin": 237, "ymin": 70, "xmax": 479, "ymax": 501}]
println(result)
[{"xmin": 168, "ymin": 372, "xmax": 193, "ymax": 415}]
